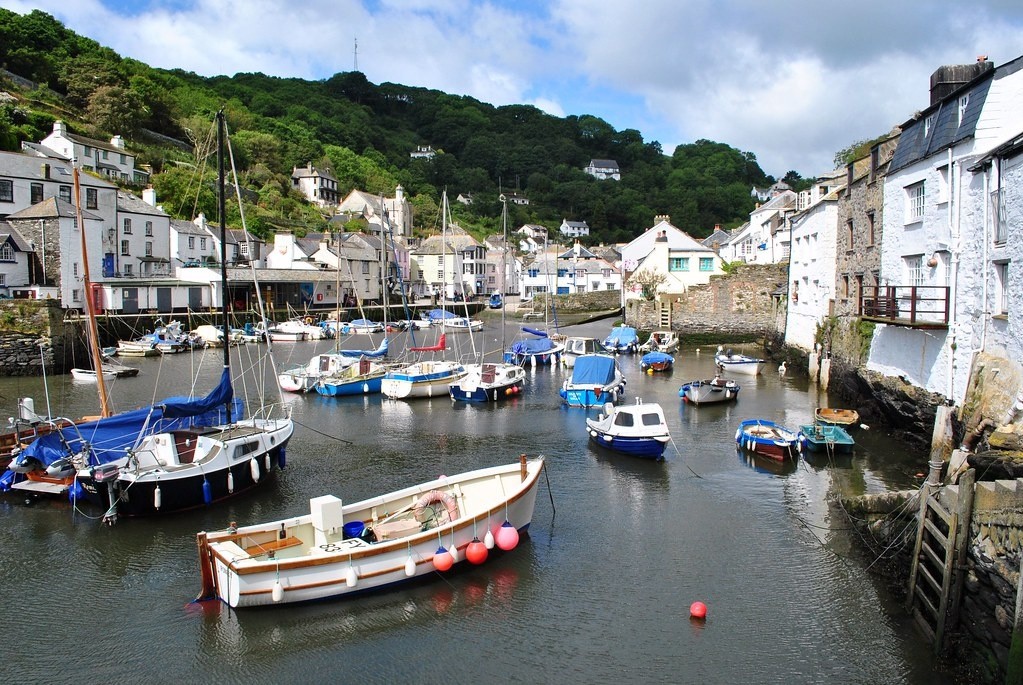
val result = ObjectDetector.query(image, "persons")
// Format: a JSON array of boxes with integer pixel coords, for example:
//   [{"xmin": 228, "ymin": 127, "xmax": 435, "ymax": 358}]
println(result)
[
  {"xmin": 434, "ymin": 290, "xmax": 447, "ymax": 305},
  {"xmin": 454, "ymin": 289, "xmax": 473, "ymax": 303}
]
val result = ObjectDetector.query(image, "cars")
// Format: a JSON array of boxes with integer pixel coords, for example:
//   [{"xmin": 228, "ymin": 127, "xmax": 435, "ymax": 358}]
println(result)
[{"xmin": 489, "ymin": 299, "xmax": 502, "ymax": 308}]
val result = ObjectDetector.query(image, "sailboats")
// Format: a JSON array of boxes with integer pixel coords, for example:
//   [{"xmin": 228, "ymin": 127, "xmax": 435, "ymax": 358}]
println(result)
[
  {"xmin": 59, "ymin": 111, "xmax": 294, "ymax": 517},
  {"xmin": 0, "ymin": 169, "xmax": 142, "ymax": 478},
  {"xmin": 381, "ymin": 191, "xmax": 481, "ymax": 399},
  {"xmin": 447, "ymin": 202, "xmax": 527, "ymax": 403},
  {"xmin": 313, "ymin": 193, "xmax": 420, "ymax": 396},
  {"xmin": 502, "ymin": 237, "xmax": 564, "ymax": 364},
  {"xmin": 277, "ymin": 227, "xmax": 376, "ymax": 391}
]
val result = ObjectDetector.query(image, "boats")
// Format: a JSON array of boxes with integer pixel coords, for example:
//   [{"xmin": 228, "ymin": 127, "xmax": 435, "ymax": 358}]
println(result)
[
  {"xmin": 185, "ymin": 455, "xmax": 545, "ymax": 613},
  {"xmin": 115, "ymin": 315, "xmax": 383, "ymax": 358},
  {"xmin": 71, "ymin": 362, "xmax": 140, "ymax": 381},
  {"xmin": 561, "ymin": 322, "xmax": 679, "ymax": 371},
  {"xmin": 585, "ymin": 396, "xmax": 672, "ymax": 456},
  {"xmin": 563, "ymin": 353, "xmax": 626, "ymax": 407},
  {"xmin": 388, "ymin": 308, "xmax": 484, "ymax": 334},
  {"xmin": 0, "ymin": 395, "xmax": 244, "ymax": 495},
  {"xmin": 734, "ymin": 408, "xmax": 861, "ymax": 462},
  {"xmin": 714, "ymin": 346, "xmax": 766, "ymax": 376},
  {"xmin": 735, "ymin": 444, "xmax": 799, "ymax": 479},
  {"xmin": 680, "ymin": 374, "xmax": 740, "ymax": 405}
]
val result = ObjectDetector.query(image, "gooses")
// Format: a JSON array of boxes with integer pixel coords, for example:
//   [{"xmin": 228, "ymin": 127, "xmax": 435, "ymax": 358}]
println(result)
[
  {"xmin": 779, "ymin": 368, "xmax": 786, "ymax": 381},
  {"xmin": 778, "ymin": 361, "xmax": 786, "ymax": 371}
]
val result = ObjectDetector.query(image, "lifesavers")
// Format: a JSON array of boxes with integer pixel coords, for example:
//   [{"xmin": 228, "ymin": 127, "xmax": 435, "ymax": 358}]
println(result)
[
  {"xmin": 415, "ymin": 491, "xmax": 459, "ymax": 529},
  {"xmin": 306, "ymin": 317, "xmax": 312, "ymax": 324}
]
